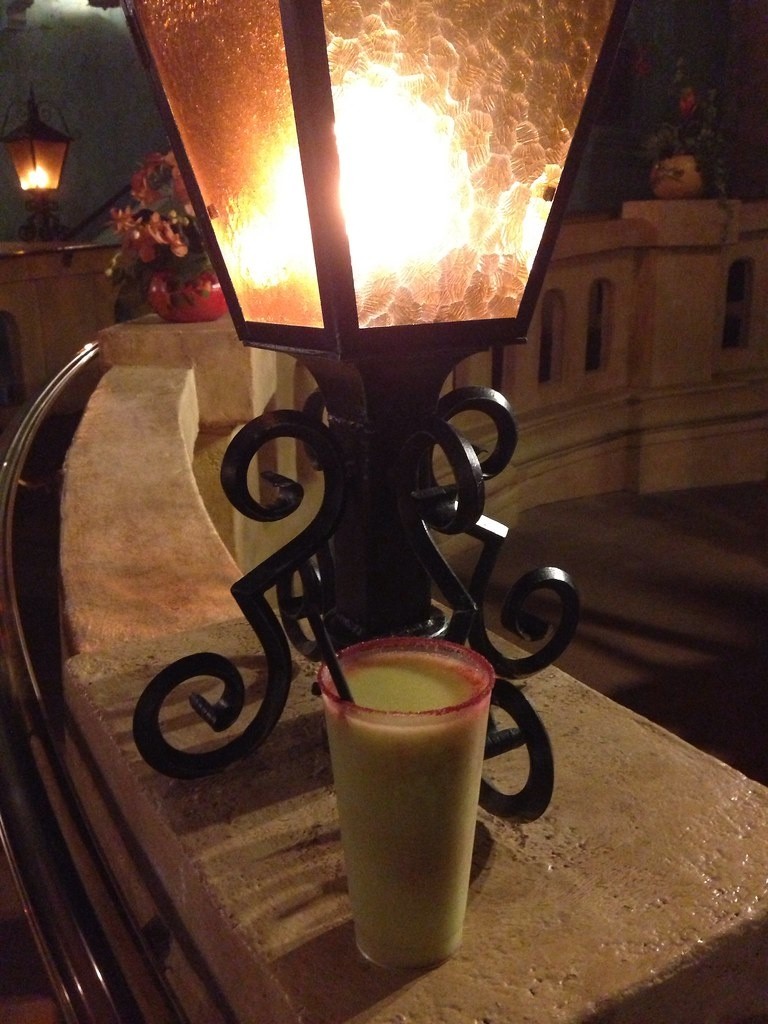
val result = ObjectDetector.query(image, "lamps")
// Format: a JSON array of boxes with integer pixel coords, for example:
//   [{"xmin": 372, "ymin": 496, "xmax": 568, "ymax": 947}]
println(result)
[
  {"xmin": 123, "ymin": 1, "xmax": 616, "ymax": 830},
  {"xmin": 1, "ymin": 83, "xmax": 84, "ymax": 246}
]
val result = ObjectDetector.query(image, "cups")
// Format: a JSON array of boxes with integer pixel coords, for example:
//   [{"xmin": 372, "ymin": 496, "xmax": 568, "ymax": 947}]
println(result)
[{"xmin": 318, "ymin": 636, "xmax": 496, "ymax": 971}]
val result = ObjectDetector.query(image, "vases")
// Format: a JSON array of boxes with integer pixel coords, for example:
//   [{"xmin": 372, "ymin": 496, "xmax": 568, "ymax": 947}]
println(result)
[
  {"xmin": 652, "ymin": 150, "xmax": 703, "ymax": 201},
  {"xmin": 145, "ymin": 262, "xmax": 225, "ymax": 322}
]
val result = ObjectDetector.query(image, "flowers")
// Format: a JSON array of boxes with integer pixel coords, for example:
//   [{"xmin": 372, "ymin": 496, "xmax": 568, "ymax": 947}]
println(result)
[
  {"xmin": 107, "ymin": 147, "xmax": 229, "ymax": 299},
  {"xmin": 634, "ymin": 47, "xmax": 733, "ymax": 202}
]
